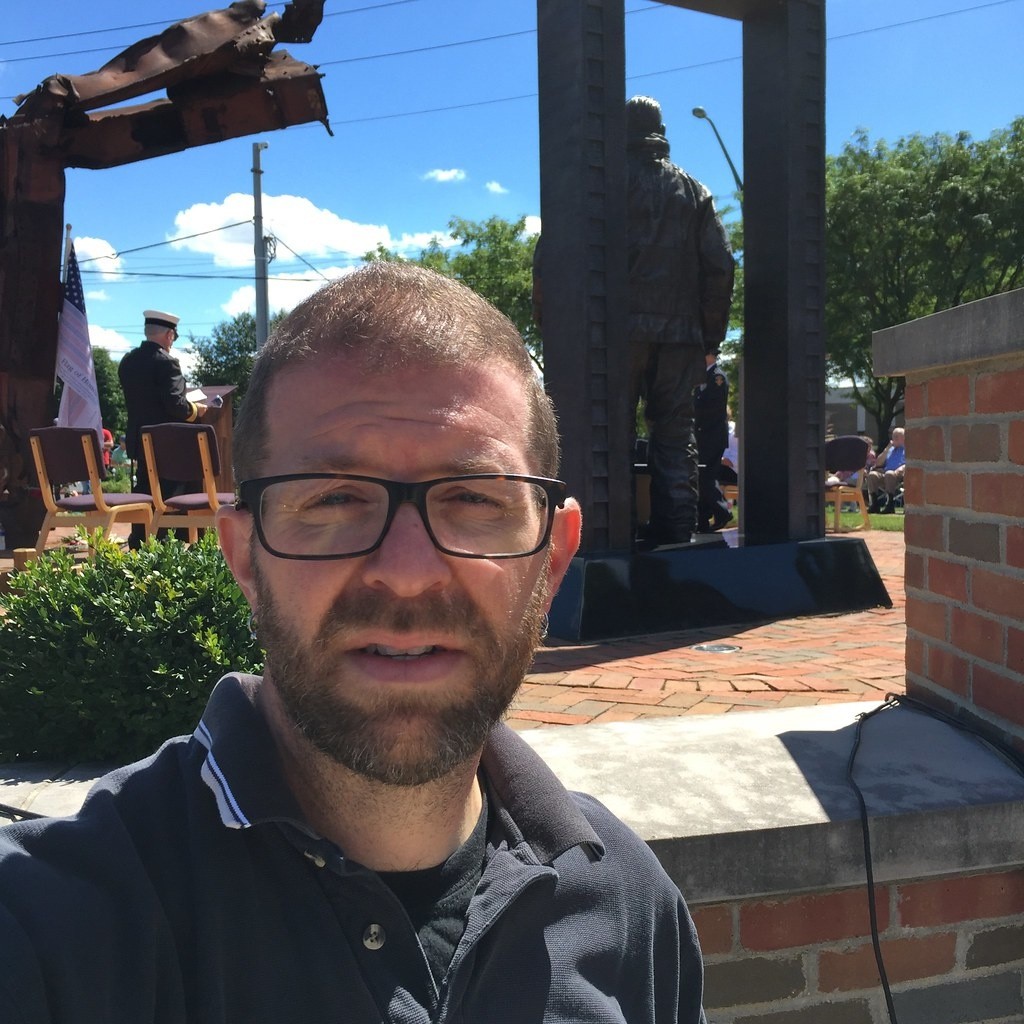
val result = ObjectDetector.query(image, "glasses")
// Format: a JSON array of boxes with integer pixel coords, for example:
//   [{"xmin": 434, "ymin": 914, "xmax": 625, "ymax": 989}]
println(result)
[{"xmin": 232, "ymin": 474, "xmax": 567, "ymax": 560}]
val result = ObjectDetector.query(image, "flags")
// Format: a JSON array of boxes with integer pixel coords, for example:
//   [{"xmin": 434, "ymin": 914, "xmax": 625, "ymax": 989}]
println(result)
[{"xmin": 55, "ymin": 232, "xmax": 106, "ymax": 453}]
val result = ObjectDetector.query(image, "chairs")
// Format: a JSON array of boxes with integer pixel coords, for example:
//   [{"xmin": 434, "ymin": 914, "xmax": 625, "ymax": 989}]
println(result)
[
  {"xmin": 709, "ymin": 484, "xmax": 737, "ymax": 526},
  {"xmin": 26, "ymin": 426, "xmax": 156, "ymax": 572},
  {"xmin": 824, "ymin": 435, "xmax": 873, "ymax": 534}
]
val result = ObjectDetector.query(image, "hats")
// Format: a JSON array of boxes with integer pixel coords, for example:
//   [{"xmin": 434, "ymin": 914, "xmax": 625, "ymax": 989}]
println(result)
[{"xmin": 143, "ymin": 308, "xmax": 179, "ymax": 330}]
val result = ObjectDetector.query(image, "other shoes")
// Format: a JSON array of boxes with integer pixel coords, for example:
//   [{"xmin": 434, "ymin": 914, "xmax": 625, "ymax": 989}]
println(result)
[
  {"xmin": 865, "ymin": 501, "xmax": 896, "ymax": 514},
  {"xmin": 696, "ymin": 511, "xmax": 736, "ymax": 532}
]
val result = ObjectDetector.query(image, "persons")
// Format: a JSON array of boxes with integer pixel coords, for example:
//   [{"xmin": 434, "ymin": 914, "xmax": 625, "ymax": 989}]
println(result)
[
  {"xmin": 49, "ymin": 418, "xmax": 71, "ymax": 503},
  {"xmin": 81, "ymin": 417, "xmax": 115, "ymax": 495},
  {"xmin": 715, "ymin": 402, "xmax": 738, "ymax": 486},
  {"xmin": 866, "ymin": 427, "xmax": 906, "ymax": 515},
  {"xmin": 110, "ymin": 434, "xmax": 138, "ymax": 488},
  {"xmin": 530, "ymin": 93, "xmax": 737, "ymax": 547},
  {"xmin": 690, "ymin": 347, "xmax": 734, "ymax": 535},
  {"xmin": 118, "ymin": 309, "xmax": 208, "ymax": 554},
  {"xmin": 832, "ymin": 436, "xmax": 876, "ymax": 514},
  {"xmin": 2, "ymin": 260, "xmax": 714, "ymax": 1024}
]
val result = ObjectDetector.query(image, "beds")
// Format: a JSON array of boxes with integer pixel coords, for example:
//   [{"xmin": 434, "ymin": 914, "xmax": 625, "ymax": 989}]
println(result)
[{"xmin": 141, "ymin": 423, "xmax": 237, "ymax": 547}]
